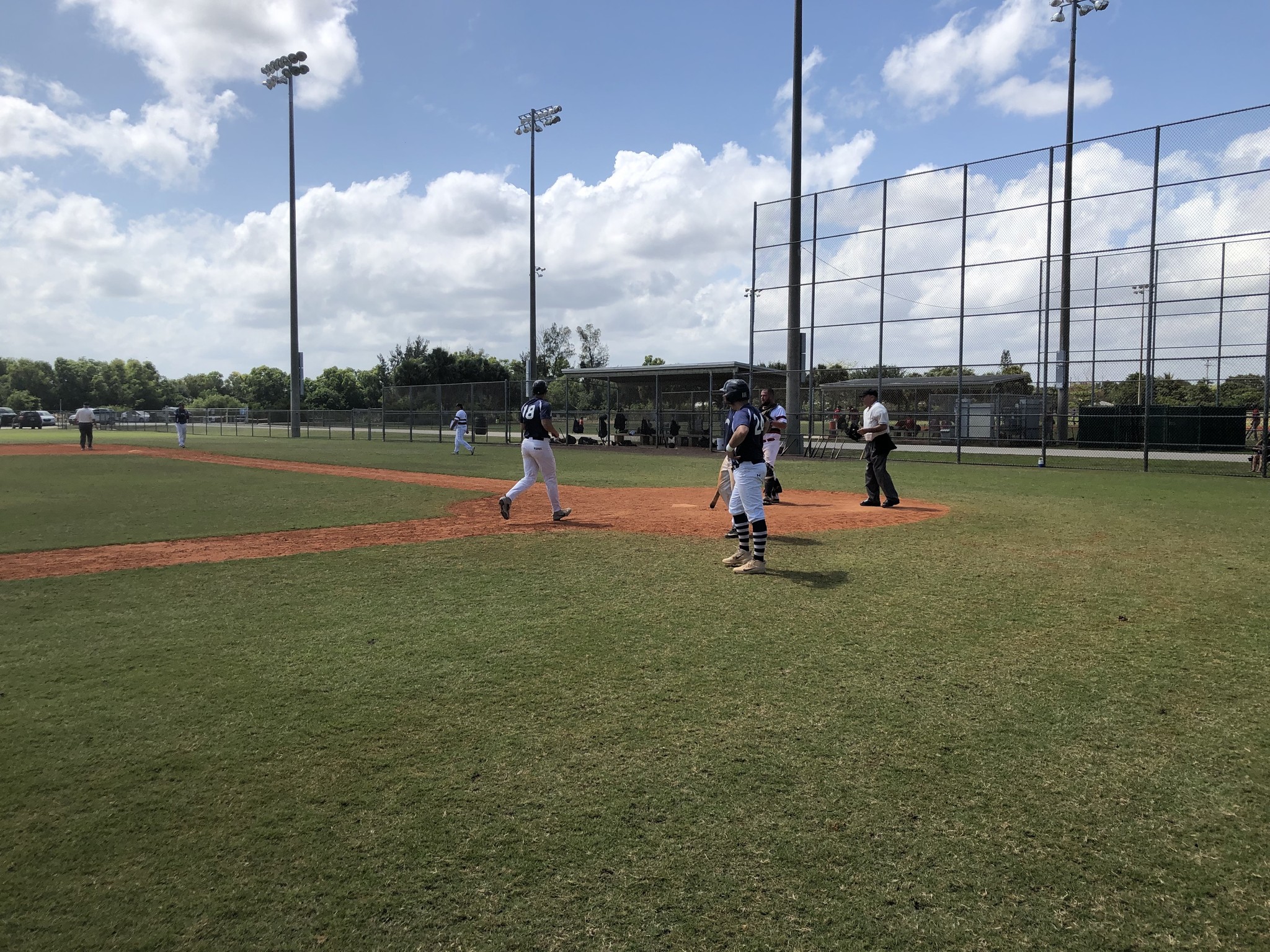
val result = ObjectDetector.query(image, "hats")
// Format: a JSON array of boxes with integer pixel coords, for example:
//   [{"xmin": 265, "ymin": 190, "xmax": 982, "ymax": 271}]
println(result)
[
  {"xmin": 858, "ymin": 388, "xmax": 878, "ymax": 397},
  {"xmin": 533, "ymin": 380, "xmax": 547, "ymax": 395},
  {"xmin": 454, "ymin": 403, "xmax": 463, "ymax": 406},
  {"xmin": 83, "ymin": 402, "xmax": 90, "ymax": 406},
  {"xmin": 178, "ymin": 402, "xmax": 184, "ymax": 406}
]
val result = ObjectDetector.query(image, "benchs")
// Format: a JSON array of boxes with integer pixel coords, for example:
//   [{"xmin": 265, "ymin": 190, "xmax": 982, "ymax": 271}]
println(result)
[
  {"xmin": 825, "ymin": 429, "xmax": 941, "ymax": 437},
  {"xmin": 610, "ymin": 433, "xmax": 724, "ymax": 447}
]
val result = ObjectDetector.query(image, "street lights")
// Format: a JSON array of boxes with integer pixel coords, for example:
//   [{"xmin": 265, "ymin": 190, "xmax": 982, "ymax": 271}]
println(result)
[
  {"xmin": 261, "ymin": 56, "xmax": 310, "ymax": 439},
  {"xmin": 1134, "ymin": 284, "xmax": 1150, "ymax": 406},
  {"xmin": 1051, "ymin": 0, "xmax": 1110, "ymax": 442},
  {"xmin": 514, "ymin": 106, "xmax": 565, "ymax": 400}
]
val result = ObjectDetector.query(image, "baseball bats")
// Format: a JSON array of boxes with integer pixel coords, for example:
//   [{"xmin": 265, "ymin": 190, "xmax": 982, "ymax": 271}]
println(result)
[{"xmin": 710, "ymin": 491, "xmax": 720, "ymax": 509}]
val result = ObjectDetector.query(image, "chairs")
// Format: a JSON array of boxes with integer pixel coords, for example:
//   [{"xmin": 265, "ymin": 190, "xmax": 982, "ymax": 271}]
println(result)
[{"xmin": 1248, "ymin": 450, "xmax": 1270, "ymax": 472}]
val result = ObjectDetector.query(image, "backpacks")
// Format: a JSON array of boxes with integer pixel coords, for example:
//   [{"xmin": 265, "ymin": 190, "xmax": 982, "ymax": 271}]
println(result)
[{"xmin": 565, "ymin": 413, "xmax": 718, "ymax": 448}]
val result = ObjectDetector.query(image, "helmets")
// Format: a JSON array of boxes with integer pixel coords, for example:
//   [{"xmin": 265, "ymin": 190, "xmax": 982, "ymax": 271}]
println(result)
[{"xmin": 719, "ymin": 379, "xmax": 749, "ymax": 402}]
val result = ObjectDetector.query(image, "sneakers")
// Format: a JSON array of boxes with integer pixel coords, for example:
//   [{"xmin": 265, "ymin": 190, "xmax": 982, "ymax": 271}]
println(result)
[
  {"xmin": 733, "ymin": 559, "xmax": 766, "ymax": 574},
  {"xmin": 860, "ymin": 498, "xmax": 899, "ymax": 508},
  {"xmin": 451, "ymin": 451, "xmax": 459, "ymax": 454},
  {"xmin": 723, "ymin": 549, "xmax": 752, "ymax": 566},
  {"xmin": 470, "ymin": 446, "xmax": 475, "ymax": 456},
  {"xmin": 499, "ymin": 495, "xmax": 511, "ymax": 520},
  {"xmin": 552, "ymin": 508, "xmax": 571, "ymax": 520},
  {"xmin": 763, "ymin": 498, "xmax": 779, "ymax": 505}
]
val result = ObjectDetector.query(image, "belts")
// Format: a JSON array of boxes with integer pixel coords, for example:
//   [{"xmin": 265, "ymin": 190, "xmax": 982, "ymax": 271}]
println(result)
[
  {"xmin": 525, "ymin": 436, "xmax": 544, "ymax": 441},
  {"xmin": 763, "ymin": 438, "xmax": 775, "ymax": 442},
  {"xmin": 732, "ymin": 457, "xmax": 750, "ymax": 469},
  {"xmin": 457, "ymin": 426, "xmax": 466, "ymax": 428},
  {"xmin": 176, "ymin": 422, "xmax": 185, "ymax": 424}
]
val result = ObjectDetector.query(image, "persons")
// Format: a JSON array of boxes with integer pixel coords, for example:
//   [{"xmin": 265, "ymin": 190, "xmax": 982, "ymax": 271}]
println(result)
[
  {"xmin": 905, "ymin": 414, "xmax": 921, "ymax": 437},
  {"xmin": 75, "ymin": 402, "xmax": 99, "ymax": 450},
  {"xmin": 1246, "ymin": 404, "xmax": 1260, "ymax": 440},
  {"xmin": 450, "ymin": 403, "xmax": 475, "ymax": 455},
  {"xmin": 499, "ymin": 380, "xmax": 571, "ymax": 520},
  {"xmin": 579, "ymin": 417, "xmax": 584, "ymax": 426},
  {"xmin": 857, "ymin": 389, "xmax": 900, "ymax": 508},
  {"xmin": 717, "ymin": 379, "xmax": 767, "ymax": 573},
  {"xmin": 174, "ymin": 402, "xmax": 189, "ymax": 448},
  {"xmin": 758, "ymin": 388, "xmax": 787, "ymax": 505},
  {"xmin": 833, "ymin": 405, "xmax": 860, "ymax": 433}
]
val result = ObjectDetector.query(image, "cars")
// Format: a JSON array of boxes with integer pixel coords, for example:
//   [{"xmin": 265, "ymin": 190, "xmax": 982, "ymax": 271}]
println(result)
[
  {"xmin": 150, "ymin": 406, "xmax": 185, "ymax": 423},
  {"xmin": 13, "ymin": 410, "xmax": 43, "ymax": 430},
  {"xmin": 36, "ymin": 409, "xmax": 55, "ymax": 425},
  {"xmin": 0, "ymin": 407, "xmax": 17, "ymax": 426},
  {"xmin": 121, "ymin": 410, "xmax": 151, "ymax": 422},
  {"xmin": 68, "ymin": 407, "xmax": 117, "ymax": 426}
]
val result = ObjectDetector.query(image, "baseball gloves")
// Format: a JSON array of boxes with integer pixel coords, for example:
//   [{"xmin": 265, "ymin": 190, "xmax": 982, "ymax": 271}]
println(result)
[{"xmin": 844, "ymin": 423, "xmax": 862, "ymax": 442}]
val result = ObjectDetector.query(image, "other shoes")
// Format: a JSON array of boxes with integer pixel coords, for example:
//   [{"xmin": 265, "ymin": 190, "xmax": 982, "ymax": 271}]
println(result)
[
  {"xmin": 724, "ymin": 529, "xmax": 739, "ymax": 539},
  {"xmin": 82, "ymin": 447, "xmax": 92, "ymax": 450},
  {"xmin": 181, "ymin": 445, "xmax": 186, "ymax": 448}
]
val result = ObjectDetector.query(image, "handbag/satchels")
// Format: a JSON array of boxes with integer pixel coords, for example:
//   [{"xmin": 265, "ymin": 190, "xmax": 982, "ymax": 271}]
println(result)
[
  {"xmin": 829, "ymin": 421, "xmax": 838, "ymax": 438},
  {"xmin": 874, "ymin": 433, "xmax": 897, "ymax": 455}
]
